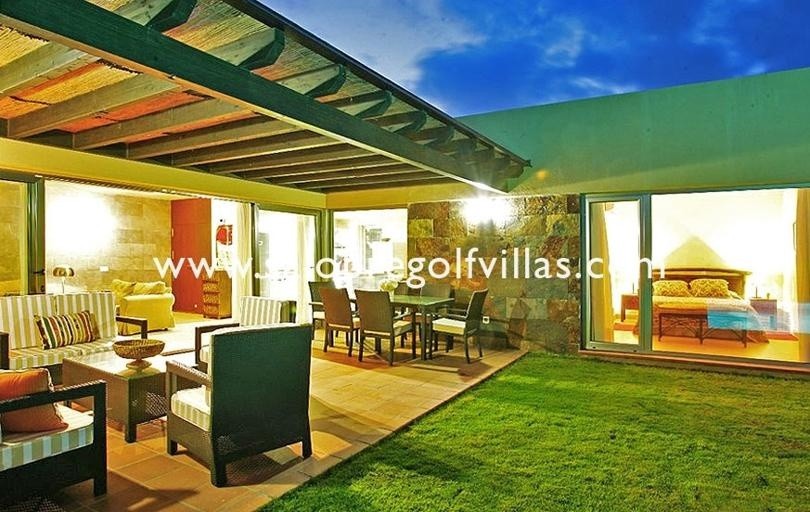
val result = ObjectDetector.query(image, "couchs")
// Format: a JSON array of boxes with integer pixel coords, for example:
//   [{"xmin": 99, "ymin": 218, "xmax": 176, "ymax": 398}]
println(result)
[
  {"xmin": 103, "ymin": 282, "xmax": 176, "ymax": 334},
  {"xmin": 2, "ymin": 292, "xmax": 151, "ymax": 385}
]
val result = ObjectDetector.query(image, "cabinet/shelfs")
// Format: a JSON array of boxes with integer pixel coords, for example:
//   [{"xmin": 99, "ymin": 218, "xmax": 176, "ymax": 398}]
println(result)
[{"xmin": 201, "ymin": 268, "xmax": 232, "ymax": 319}]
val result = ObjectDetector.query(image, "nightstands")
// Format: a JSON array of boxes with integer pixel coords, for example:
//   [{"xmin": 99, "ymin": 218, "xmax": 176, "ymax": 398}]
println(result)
[
  {"xmin": 619, "ymin": 294, "xmax": 641, "ymax": 321},
  {"xmin": 748, "ymin": 296, "xmax": 777, "ymax": 331}
]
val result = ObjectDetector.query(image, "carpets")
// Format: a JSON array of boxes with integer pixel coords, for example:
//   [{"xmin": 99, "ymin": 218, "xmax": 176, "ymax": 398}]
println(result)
[{"xmin": 612, "ymin": 319, "xmax": 798, "ymax": 341}]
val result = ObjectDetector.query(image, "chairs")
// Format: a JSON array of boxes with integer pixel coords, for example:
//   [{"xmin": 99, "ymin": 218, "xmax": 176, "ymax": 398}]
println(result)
[
  {"xmin": 161, "ymin": 321, "xmax": 313, "ymax": 484},
  {"xmin": 1, "ymin": 367, "xmax": 109, "ymax": 510},
  {"xmin": 194, "ymin": 293, "xmax": 298, "ymax": 418},
  {"xmin": 305, "ymin": 274, "xmax": 489, "ymax": 366}
]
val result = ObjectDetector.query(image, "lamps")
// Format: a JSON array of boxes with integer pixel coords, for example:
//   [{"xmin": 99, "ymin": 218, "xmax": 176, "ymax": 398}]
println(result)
[{"xmin": 50, "ymin": 264, "xmax": 75, "ymax": 294}]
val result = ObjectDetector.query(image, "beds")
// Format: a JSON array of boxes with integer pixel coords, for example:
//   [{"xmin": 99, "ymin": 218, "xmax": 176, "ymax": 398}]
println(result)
[
  {"xmin": 59, "ymin": 348, "xmax": 200, "ymax": 442},
  {"xmin": 632, "ymin": 264, "xmax": 771, "ymax": 344}
]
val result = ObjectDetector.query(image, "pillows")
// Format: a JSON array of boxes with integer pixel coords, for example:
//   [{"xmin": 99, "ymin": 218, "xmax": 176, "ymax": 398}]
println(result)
[
  {"xmin": 105, "ymin": 277, "xmax": 134, "ymax": 303},
  {"xmin": 651, "ymin": 279, "xmax": 690, "ymax": 297},
  {"xmin": 689, "ymin": 279, "xmax": 732, "ymax": 296},
  {"xmin": 34, "ymin": 309, "xmax": 104, "ymax": 352},
  {"xmin": 1, "ymin": 368, "xmax": 70, "ymax": 431},
  {"xmin": 132, "ymin": 280, "xmax": 165, "ymax": 297}
]
